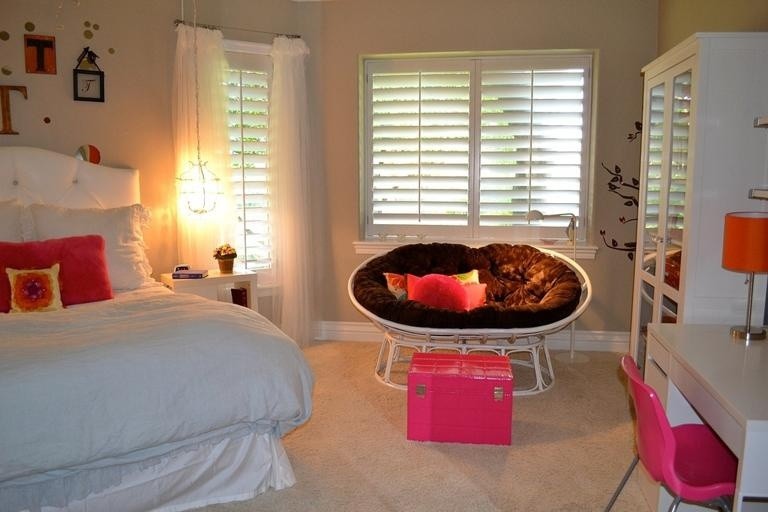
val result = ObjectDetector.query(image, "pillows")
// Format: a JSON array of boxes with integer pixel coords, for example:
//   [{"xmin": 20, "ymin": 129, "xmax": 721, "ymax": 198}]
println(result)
[
  {"xmin": 0, "ymin": 235, "xmax": 115, "ymax": 313},
  {"xmin": 382, "ymin": 270, "xmax": 488, "ymax": 313},
  {"xmin": 5, "ymin": 263, "xmax": 65, "ymax": 314},
  {"xmin": 23, "ymin": 204, "xmax": 163, "ymax": 289},
  {"xmin": 0, "ymin": 204, "xmax": 24, "ymax": 241}
]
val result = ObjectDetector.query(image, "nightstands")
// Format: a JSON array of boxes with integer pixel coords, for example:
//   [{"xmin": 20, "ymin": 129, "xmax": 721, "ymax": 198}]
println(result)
[{"xmin": 162, "ymin": 273, "xmax": 259, "ymax": 314}]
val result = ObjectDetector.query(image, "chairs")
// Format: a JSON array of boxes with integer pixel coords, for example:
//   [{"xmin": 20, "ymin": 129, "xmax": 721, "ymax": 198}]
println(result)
[{"xmin": 604, "ymin": 355, "xmax": 739, "ymax": 512}]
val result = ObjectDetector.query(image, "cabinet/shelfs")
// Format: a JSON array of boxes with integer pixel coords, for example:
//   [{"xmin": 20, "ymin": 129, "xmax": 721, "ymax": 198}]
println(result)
[{"xmin": 628, "ymin": 32, "xmax": 768, "ymax": 428}]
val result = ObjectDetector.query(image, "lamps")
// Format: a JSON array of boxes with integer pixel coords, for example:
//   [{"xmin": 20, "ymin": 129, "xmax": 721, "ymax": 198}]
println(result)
[
  {"xmin": 525, "ymin": 210, "xmax": 590, "ymax": 366},
  {"xmin": 721, "ymin": 212, "xmax": 768, "ymax": 340},
  {"xmin": 176, "ymin": 0, "xmax": 225, "ymax": 216}
]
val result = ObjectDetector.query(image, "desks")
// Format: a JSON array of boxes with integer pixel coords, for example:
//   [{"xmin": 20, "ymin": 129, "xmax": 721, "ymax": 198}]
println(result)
[{"xmin": 639, "ymin": 322, "xmax": 768, "ymax": 512}]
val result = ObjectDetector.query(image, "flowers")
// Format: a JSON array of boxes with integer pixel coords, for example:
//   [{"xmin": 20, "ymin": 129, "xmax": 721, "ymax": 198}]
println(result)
[{"xmin": 213, "ymin": 243, "xmax": 237, "ymax": 259}]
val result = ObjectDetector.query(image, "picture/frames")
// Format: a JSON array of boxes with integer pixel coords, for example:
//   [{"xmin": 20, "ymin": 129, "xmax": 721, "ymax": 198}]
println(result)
[{"xmin": 73, "ymin": 69, "xmax": 104, "ymax": 102}]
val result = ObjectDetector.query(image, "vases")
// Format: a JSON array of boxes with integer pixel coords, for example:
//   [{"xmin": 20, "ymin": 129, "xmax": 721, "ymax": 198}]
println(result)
[{"xmin": 217, "ymin": 258, "xmax": 234, "ymax": 273}]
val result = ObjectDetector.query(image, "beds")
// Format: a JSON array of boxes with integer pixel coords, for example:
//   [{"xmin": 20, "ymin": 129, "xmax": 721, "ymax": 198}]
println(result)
[{"xmin": 0, "ymin": 146, "xmax": 279, "ymax": 512}]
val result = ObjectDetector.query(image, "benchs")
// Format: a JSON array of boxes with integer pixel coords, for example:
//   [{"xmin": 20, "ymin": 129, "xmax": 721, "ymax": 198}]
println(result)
[{"xmin": 347, "ymin": 243, "xmax": 593, "ymax": 397}]
